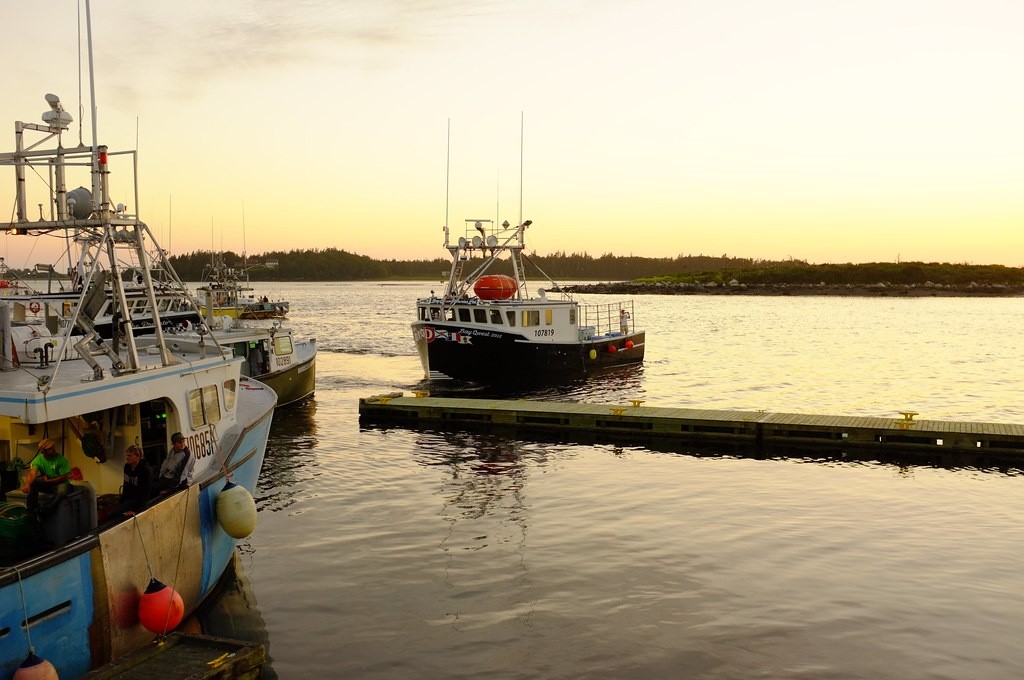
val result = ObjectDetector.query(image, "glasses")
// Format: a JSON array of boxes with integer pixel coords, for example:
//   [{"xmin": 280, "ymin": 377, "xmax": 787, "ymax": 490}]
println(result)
[{"xmin": 125, "ymin": 450, "xmax": 133, "ymax": 453}]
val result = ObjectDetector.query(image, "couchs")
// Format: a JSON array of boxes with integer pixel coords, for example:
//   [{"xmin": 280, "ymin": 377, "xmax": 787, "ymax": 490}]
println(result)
[{"xmin": 10, "ymin": 325, "xmax": 89, "ymax": 362}]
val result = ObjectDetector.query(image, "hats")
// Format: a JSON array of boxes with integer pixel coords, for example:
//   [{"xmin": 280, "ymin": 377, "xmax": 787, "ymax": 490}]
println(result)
[
  {"xmin": 621, "ymin": 310, "xmax": 625, "ymax": 313},
  {"xmin": 171, "ymin": 432, "xmax": 188, "ymax": 443},
  {"xmin": 38, "ymin": 439, "xmax": 56, "ymax": 451},
  {"xmin": 127, "ymin": 445, "xmax": 142, "ymax": 458}
]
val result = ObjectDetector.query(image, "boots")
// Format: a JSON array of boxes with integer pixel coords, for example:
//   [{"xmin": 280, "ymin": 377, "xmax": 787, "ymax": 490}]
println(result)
[
  {"xmin": 22, "ymin": 494, "xmax": 41, "ymax": 516},
  {"xmin": 36, "ymin": 506, "xmax": 52, "ymax": 523}
]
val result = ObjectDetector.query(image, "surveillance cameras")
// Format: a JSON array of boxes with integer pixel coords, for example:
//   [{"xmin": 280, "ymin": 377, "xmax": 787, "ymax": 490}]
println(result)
[{"xmin": 475, "ymin": 222, "xmax": 482, "ymax": 230}]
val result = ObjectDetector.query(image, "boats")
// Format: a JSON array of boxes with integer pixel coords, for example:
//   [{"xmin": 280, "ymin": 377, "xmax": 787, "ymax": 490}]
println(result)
[
  {"xmin": 0, "ymin": 0, "xmax": 320, "ymax": 680},
  {"xmin": 410, "ymin": 108, "xmax": 646, "ymax": 392}
]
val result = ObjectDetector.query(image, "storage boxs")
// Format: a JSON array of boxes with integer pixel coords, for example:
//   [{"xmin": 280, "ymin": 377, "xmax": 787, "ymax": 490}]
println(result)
[{"xmin": 0, "ymin": 502, "xmax": 30, "ymax": 562}]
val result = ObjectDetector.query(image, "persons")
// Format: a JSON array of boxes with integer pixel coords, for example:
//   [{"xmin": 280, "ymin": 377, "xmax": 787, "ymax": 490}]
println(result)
[
  {"xmin": 120, "ymin": 444, "xmax": 156, "ymax": 512},
  {"xmin": 21, "ymin": 438, "xmax": 72, "ymax": 513},
  {"xmin": 156, "ymin": 434, "xmax": 196, "ymax": 494},
  {"xmin": 619, "ymin": 309, "xmax": 630, "ymax": 334}
]
